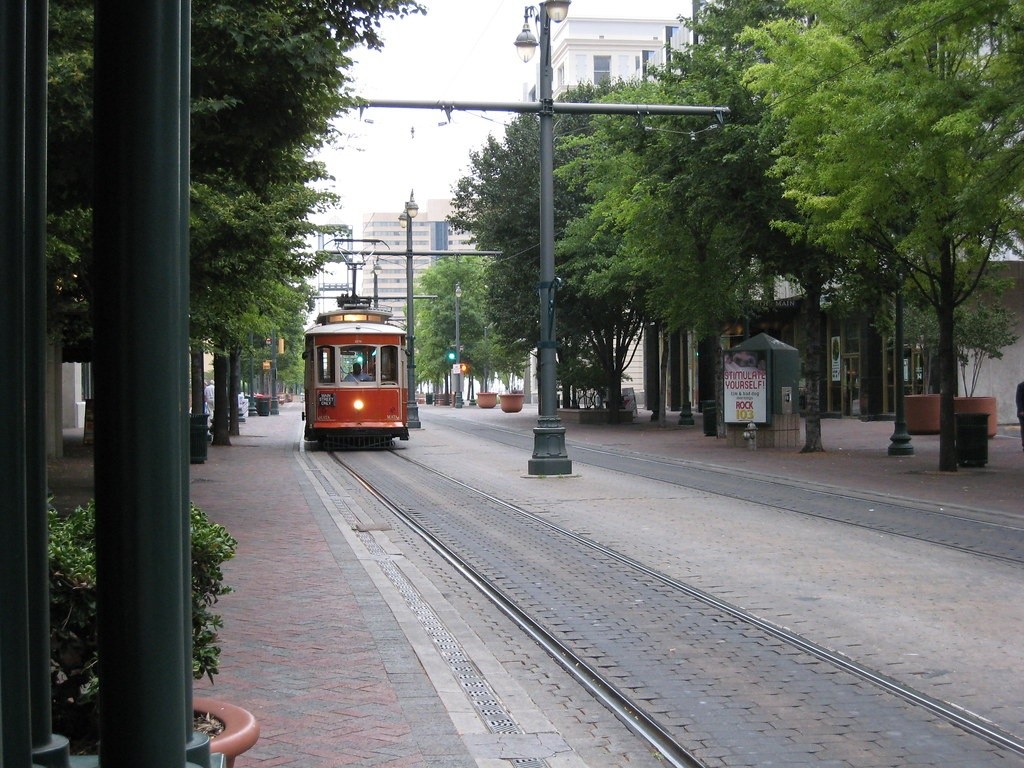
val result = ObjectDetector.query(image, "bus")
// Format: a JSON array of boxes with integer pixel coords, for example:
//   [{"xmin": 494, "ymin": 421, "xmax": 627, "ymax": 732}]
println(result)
[{"xmin": 301, "ymin": 238, "xmax": 409, "ymax": 452}]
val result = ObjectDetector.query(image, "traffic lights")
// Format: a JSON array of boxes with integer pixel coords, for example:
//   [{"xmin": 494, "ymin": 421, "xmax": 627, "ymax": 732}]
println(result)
[{"xmin": 448, "ymin": 350, "xmax": 456, "ymax": 364}]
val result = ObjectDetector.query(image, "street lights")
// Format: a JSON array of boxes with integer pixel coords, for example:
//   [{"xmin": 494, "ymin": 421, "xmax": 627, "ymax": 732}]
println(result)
[
  {"xmin": 369, "ymin": 261, "xmax": 385, "ymax": 310},
  {"xmin": 398, "ymin": 188, "xmax": 422, "ymax": 428},
  {"xmin": 454, "ymin": 281, "xmax": 464, "ymax": 408},
  {"xmin": 513, "ymin": 0, "xmax": 575, "ymax": 475}
]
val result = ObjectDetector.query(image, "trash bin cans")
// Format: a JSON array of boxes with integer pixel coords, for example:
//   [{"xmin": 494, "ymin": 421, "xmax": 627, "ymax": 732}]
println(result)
[
  {"xmin": 256, "ymin": 397, "xmax": 270, "ymax": 416},
  {"xmin": 426, "ymin": 392, "xmax": 433, "ymax": 404},
  {"xmin": 190, "ymin": 413, "xmax": 210, "ymax": 463},
  {"xmin": 954, "ymin": 412, "xmax": 992, "ymax": 465},
  {"xmin": 700, "ymin": 400, "xmax": 718, "ymax": 437}
]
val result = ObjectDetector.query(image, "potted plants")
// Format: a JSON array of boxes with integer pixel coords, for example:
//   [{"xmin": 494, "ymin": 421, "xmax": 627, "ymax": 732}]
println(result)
[
  {"xmin": 48, "ymin": 495, "xmax": 259, "ymax": 768},
  {"xmin": 872, "ymin": 306, "xmax": 940, "ymax": 433},
  {"xmin": 492, "ymin": 341, "xmax": 531, "ymax": 413},
  {"xmin": 953, "ymin": 296, "xmax": 1020, "ymax": 436},
  {"xmin": 472, "ymin": 340, "xmax": 498, "ymax": 408}
]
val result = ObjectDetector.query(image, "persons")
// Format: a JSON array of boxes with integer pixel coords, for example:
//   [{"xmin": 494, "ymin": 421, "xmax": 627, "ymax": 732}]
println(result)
[
  {"xmin": 343, "ymin": 363, "xmax": 374, "ymax": 381},
  {"xmin": 205, "ymin": 380, "xmax": 214, "ymax": 409},
  {"xmin": 1016, "ymin": 381, "xmax": 1024, "ymax": 452}
]
